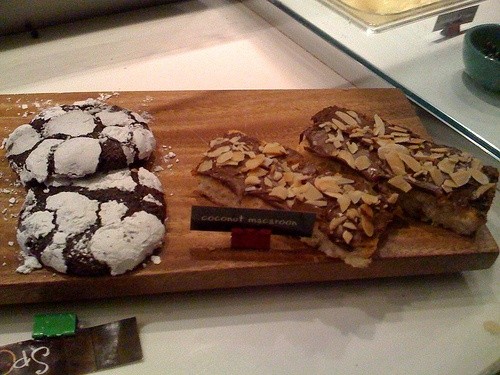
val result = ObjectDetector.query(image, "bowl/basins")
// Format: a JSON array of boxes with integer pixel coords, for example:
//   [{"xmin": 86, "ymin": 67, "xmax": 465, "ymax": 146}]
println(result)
[{"xmin": 463, "ymin": 23, "xmax": 500, "ymax": 90}]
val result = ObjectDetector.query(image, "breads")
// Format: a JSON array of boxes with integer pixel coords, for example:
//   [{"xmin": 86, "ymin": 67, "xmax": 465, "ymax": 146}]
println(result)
[
  {"xmin": 294, "ymin": 105, "xmax": 500, "ymax": 238},
  {"xmin": 189, "ymin": 131, "xmax": 401, "ymax": 269}
]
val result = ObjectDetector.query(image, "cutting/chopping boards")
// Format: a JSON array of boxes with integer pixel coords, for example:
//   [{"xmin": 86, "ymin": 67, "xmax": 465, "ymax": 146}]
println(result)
[{"xmin": 0, "ymin": 89, "xmax": 498, "ymax": 305}]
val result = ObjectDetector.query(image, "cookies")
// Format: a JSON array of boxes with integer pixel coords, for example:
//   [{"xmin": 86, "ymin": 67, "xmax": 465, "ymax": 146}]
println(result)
[
  {"xmin": 4, "ymin": 99, "xmax": 155, "ymax": 180},
  {"xmin": 16, "ymin": 166, "xmax": 167, "ymax": 277}
]
